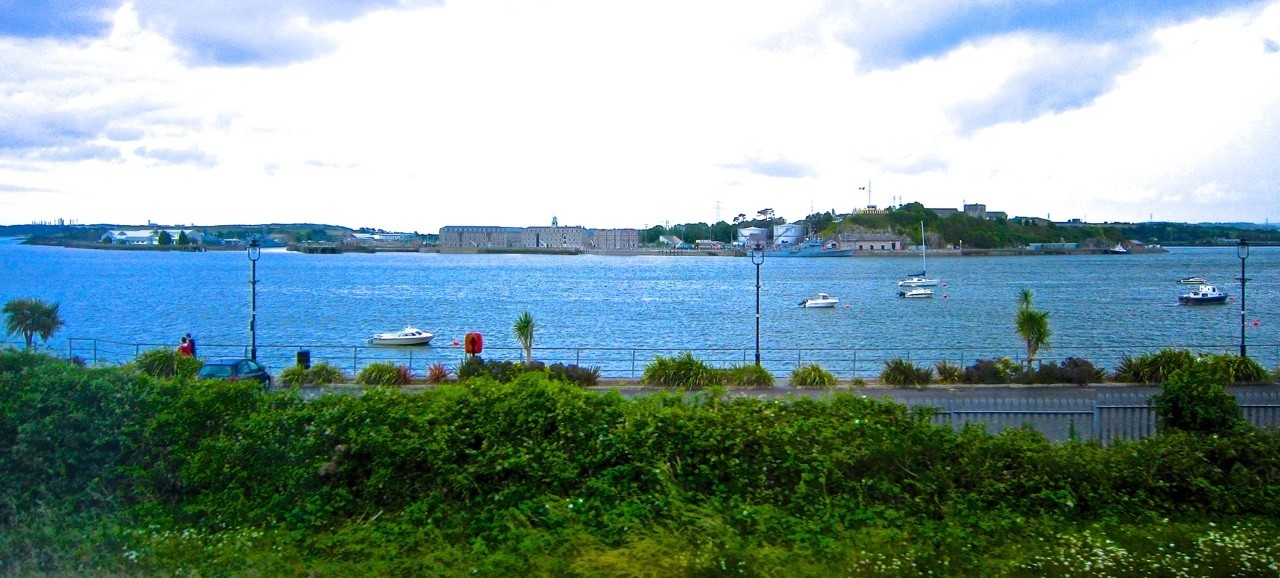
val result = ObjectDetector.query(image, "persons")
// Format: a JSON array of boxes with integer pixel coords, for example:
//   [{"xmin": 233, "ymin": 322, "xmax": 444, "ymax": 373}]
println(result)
[{"xmin": 179, "ymin": 333, "xmax": 197, "ymax": 359}]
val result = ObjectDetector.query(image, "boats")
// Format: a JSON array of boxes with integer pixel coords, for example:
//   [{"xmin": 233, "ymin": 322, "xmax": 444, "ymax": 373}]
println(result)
[
  {"xmin": 902, "ymin": 287, "xmax": 935, "ymax": 300},
  {"xmin": 373, "ymin": 326, "xmax": 434, "ymax": 345},
  {"xmin": 800, "ymin": 293, "xmax": 839, "ymax": 309},
  {"xmin": 1110, "ymin": 242, "xmax": 1129, "ymax": 255},
  {"xmin": 1177, "ymin": 282, "xmax": 1228, "ymax": 304},
  {"xmin": 746, "ymin": 232, "xmax": 855, "ymax": 258},
  {"xmin": 1179, "ymin": 277, "xmax": 1207, "ymax": 284},
  {"xmin": 898, "ymin": 220, "xmax": 938, "ymax": 286}
]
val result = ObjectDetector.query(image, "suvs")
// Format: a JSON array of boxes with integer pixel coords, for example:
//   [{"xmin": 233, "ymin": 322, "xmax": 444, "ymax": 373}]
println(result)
[{"xmin": 200, "ymin": 358, "xmax": 275, "ymax": 392}]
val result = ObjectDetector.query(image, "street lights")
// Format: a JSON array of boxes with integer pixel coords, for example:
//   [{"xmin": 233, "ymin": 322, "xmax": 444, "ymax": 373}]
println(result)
[
  {"xmin": 1234, "ymin": 234, "xmax": 1253, "ymax": 357},
  {"xmin": 751, "ymin": 241, "xmax": 764, "ymax": 364},
  {"xmin": 248, "ymin": 238, "xmax": 263, "ymax": 360}
]
